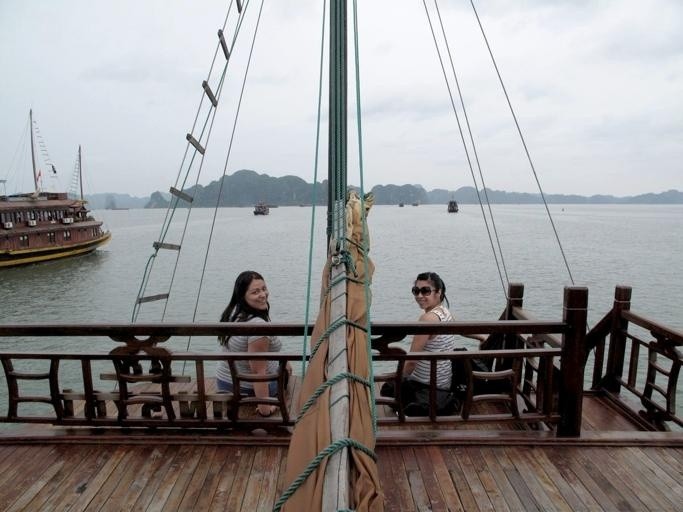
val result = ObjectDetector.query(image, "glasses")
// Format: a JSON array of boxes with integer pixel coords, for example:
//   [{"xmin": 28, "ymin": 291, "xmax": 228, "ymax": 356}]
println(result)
[{"xmin": 412, "ymin": 286, "xmax": 438, "ymax": 296}]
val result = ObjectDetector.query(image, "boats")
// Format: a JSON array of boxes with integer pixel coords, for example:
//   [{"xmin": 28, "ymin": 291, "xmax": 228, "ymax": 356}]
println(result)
[
  {"xmin": 251, "ymin": 198, "xmax": 313, "ymax": 216},
  {"xmin": 396, "ymin": 199, "xmax": 421, "ymax": 207},
  {"xmin": 0, "ymin": 100, "xmax": 112, "ymax": 267},
  {"xmin": 447, "ymin": 196, "xmax": 462, "ymax": 213}
]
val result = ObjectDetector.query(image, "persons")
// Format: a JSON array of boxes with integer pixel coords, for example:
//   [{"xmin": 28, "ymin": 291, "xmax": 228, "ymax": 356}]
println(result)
[
  {"xmin": 215, "ymin": 270, "xmax": 294, "ymax": 415},
  {"xmin": 379, "ymin": 271, "xmax": 458, "ymax": 413}
]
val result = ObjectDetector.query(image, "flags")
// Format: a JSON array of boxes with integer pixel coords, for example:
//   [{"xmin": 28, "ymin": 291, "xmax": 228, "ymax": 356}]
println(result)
[{"xmin": 29, "ymin": 172, "xmax": 44, "ymax": 198}]
